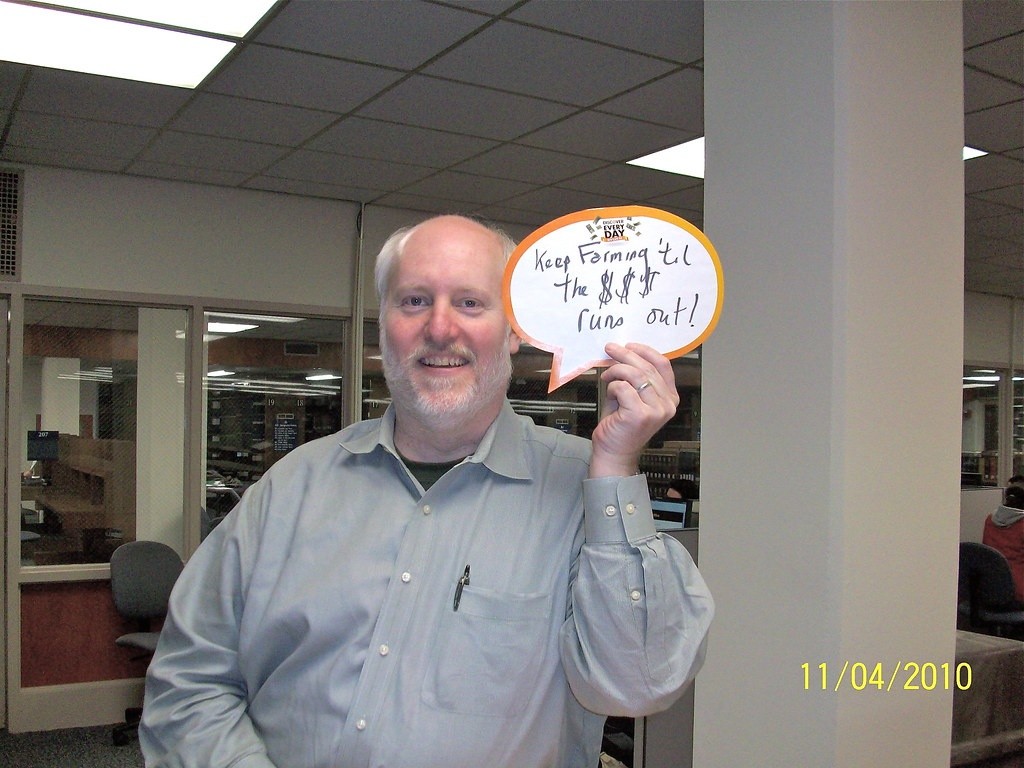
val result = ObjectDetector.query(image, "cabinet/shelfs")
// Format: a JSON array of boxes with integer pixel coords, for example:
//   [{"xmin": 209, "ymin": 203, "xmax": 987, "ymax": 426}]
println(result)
[{"xmin": 34, "ymin": 435, "xmax": 136, "ymax": 566}]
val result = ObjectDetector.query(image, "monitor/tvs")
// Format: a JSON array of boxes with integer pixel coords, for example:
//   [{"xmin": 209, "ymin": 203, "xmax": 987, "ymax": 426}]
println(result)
[{"xmin": 650, "ymin": 496, "xmax": 692, "ymax": 529}]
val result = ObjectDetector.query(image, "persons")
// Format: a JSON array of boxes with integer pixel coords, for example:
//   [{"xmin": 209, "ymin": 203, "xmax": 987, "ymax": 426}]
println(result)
[
  {"xmin": 134, "ymin": 214, "xmax": 680, "ymax": 768},
  {"xmin": 982, "ymin": 484, "xmax": 1024, "ymax": 610}
]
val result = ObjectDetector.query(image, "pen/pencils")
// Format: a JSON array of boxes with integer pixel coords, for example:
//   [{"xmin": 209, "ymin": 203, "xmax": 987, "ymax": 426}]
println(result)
[{"xmin": 453, "ymin": 564, "xmax": 470, "ymax": 611}]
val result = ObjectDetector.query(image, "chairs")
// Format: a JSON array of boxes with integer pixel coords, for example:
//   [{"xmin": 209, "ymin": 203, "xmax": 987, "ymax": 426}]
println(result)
[
  {"xmin": 958, "ymin": 541, "xmax": 1024, "ymax": 643},
  {"xmin": 111, "ymin": 541, "xmax": 186, "ymax": 747}
]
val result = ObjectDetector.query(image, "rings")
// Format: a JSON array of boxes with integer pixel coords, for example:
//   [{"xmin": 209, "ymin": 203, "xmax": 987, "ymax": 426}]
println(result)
[{"xmin": 638, "ymin": 381, "xmax": 652, "ymax": 394}]
